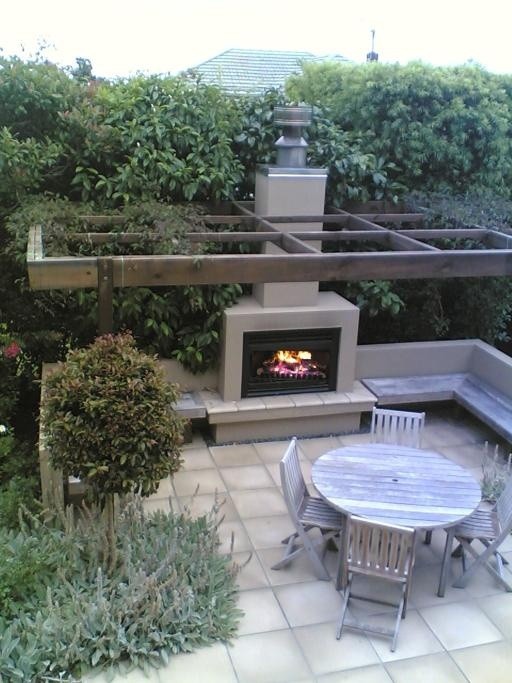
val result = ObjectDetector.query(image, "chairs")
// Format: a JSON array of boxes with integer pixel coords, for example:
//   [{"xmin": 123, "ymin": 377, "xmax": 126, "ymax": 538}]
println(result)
[
  {"xmin": 271, "ymin": 435, "xmax": 346, "ymax": 583},
  {"xmin": 422, "ymin": 478, "xmax": 512, "ymax": 597},
  {"xmin": 333, "ymin": 514, "xmax": 417, "ymax": 652},
  {"xmin": 368, "ymin": 407, "xmax": 426, "ymax": 446}
]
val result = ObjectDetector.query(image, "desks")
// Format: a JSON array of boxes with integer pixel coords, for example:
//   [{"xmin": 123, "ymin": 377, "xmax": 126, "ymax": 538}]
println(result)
[{"xmin": 310, "ymin": 444, "xmax": 484, "ymax": 597}]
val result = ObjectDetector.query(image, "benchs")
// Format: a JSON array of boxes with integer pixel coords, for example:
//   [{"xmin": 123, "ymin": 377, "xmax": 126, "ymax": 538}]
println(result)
[
  {"xmin": 361, "ymin": 372, "xmax": 512, "ymax": 445},
  {"xmin": 171, "ymin": 389, "xmax": 207, "ymax": 443}
]
